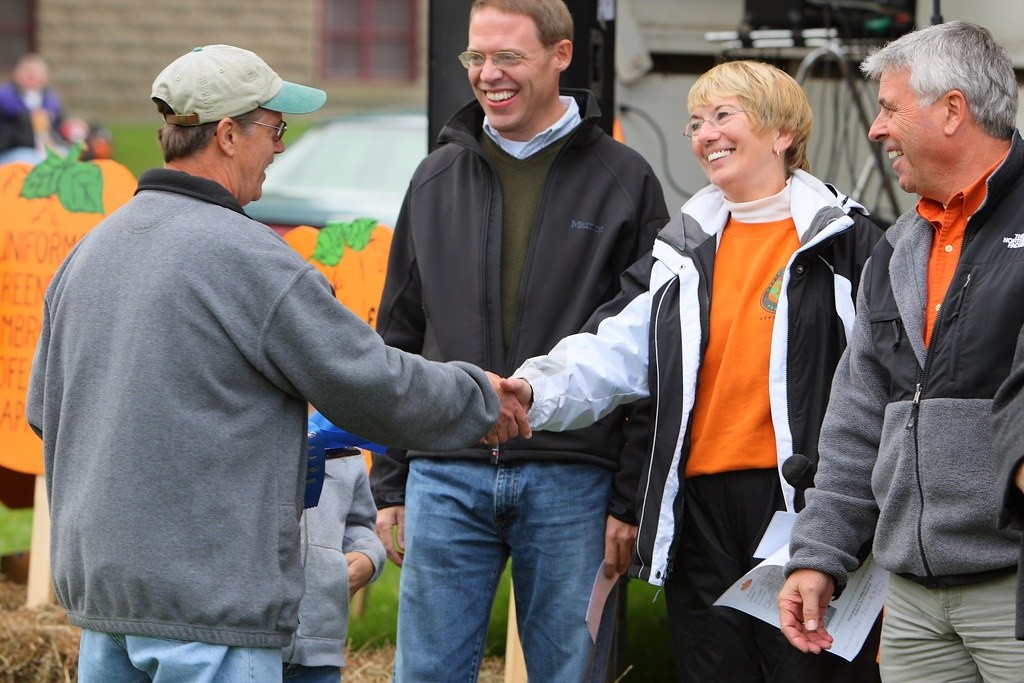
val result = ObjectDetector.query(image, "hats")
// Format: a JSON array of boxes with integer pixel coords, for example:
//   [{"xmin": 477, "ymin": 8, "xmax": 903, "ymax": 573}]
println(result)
[{"xmin": 150, "ymin": 45, "xmax": 327, "ymax": 127}]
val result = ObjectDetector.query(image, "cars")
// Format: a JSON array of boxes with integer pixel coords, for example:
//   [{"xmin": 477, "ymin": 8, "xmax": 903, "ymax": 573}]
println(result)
[{"xmin": 241, "ymin": 107, "xmax": 428, "ymax": 238}]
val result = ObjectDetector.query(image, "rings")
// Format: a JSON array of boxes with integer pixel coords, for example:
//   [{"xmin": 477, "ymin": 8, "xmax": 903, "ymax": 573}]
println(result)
[{"xmin": 801, "ymin": 622, "xmax": 805, "ymax": 625}]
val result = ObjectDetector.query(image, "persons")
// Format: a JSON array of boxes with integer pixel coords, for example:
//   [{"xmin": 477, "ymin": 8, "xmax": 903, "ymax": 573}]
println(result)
[
  {"xmin": 24, "ymin": 41, "xmax": 533, "ymax": 683},
  {"xmin": 0, "ymin": 47, "xmax": 114, "ymax": 162},
  {"xmin": 282, "ymin": 0, "xmax": 1024, "ymax": 683}
]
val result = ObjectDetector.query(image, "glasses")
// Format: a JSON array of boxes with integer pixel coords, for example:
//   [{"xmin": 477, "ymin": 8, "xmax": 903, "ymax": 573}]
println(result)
[
  {"xmin": 457, "ymin": 45, "xmax": 546, "ymax": 70},
  {"xmin": 214, "ymin": 117, "xmax": 287, "ymax": 142},
  {"xmin": 682, "ymin": 108, "xmax": 754, "ymax": 142}
]
